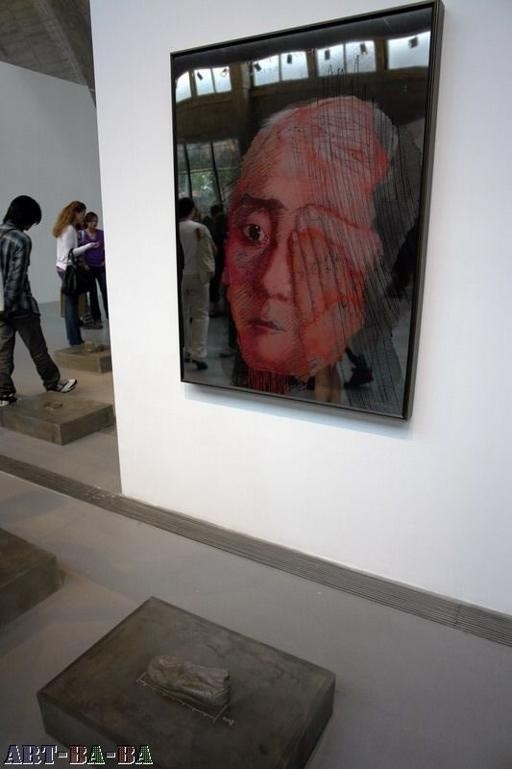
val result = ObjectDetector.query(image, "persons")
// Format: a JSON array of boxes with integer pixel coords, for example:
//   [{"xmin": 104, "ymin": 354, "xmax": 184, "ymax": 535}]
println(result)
[
  {"xmin": 1, "ymin": 196, "xmax": 108, "ymax": 408},
  {"xmin": 177, "ymin": 97, "xmax": 422, "ymax": 417}
]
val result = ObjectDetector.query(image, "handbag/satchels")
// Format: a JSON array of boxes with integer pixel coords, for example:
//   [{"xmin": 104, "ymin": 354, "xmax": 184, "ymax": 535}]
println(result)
[
  {"xmin": 58, "ymin": 248, "xmax": 94, "ymax": 318},
  {"xmin": 198, "ymin": 224, "xmax": 215, "ymax": 283}
]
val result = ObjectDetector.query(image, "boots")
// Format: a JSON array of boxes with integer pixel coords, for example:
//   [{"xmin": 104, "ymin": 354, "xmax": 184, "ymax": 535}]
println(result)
[{"xmin": 344, "ymin": 366, "xmax": 374, "ymax": 390}]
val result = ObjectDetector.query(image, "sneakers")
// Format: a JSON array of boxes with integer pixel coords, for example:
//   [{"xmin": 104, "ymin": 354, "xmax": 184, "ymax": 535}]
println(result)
[
  {"xmin": 184, "ymin": 351, "xmax": 208, "ymax": 370},
  {"xmin": 46, "ymin": 378, "xmax": 77, "ymax": 393},
  {"xmin": 1, "ymin": 392, "xmax": 17, "ymax": 407}
]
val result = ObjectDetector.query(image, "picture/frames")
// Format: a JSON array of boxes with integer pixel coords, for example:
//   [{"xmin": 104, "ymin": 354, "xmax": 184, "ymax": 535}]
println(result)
[{"xmin": 169, "ymin": 1, "xmax": 442, "ymax": 422}]
